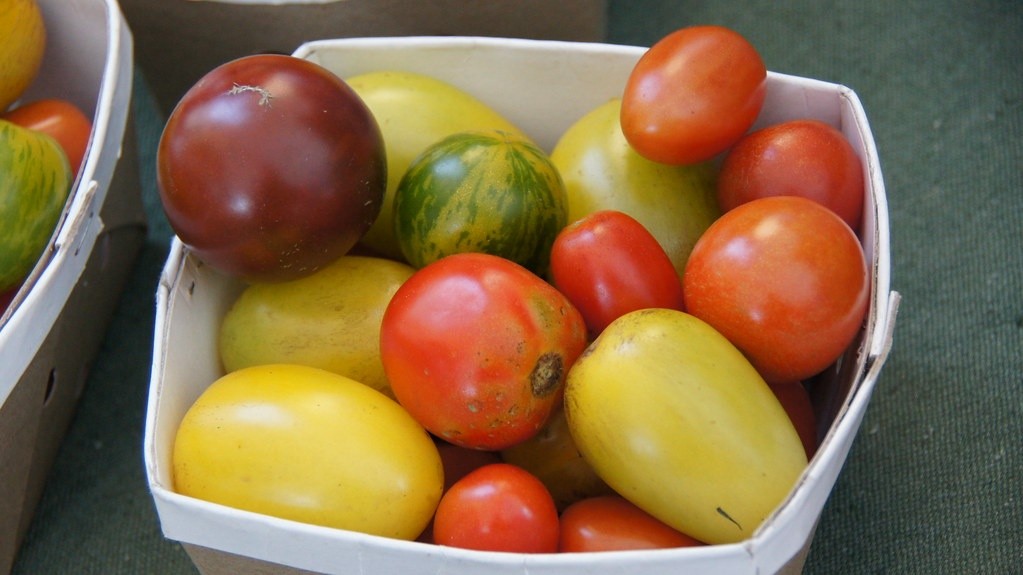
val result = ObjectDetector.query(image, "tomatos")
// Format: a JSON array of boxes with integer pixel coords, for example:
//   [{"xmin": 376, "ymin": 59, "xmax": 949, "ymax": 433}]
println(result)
[
  {"xmin": 156, "ymin": 26, "xmax": 871, "ymax": 552},
  {"xmin": 0, "ymin": 0, "xmax": 90, "ymax": 311}
]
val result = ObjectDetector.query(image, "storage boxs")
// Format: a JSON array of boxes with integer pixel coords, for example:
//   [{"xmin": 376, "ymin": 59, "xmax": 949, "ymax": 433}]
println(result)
[{"xmin": 0, "ymin": 0, "xmax": 902, "ymax": 575}]
